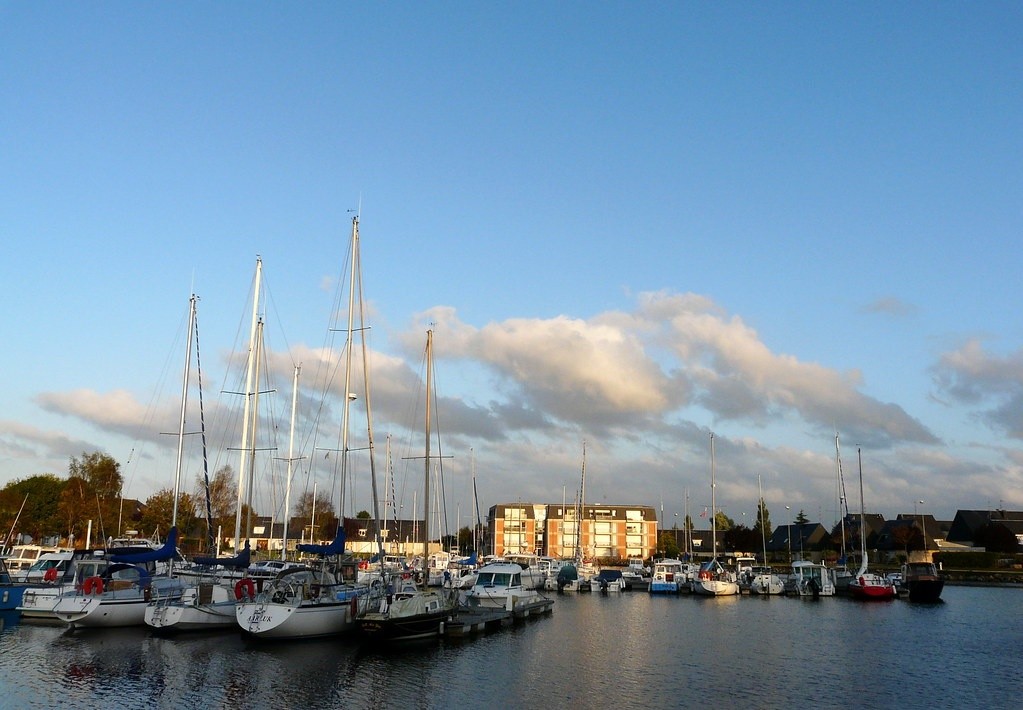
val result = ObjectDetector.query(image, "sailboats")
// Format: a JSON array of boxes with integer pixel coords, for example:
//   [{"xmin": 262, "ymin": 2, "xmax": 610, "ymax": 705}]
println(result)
[{"xmin": 0, "ymin": 184, "xmax": 950, "ymax": 644}]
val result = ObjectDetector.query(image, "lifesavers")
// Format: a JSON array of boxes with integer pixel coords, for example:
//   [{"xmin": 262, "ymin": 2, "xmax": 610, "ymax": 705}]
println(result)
[
  {"xmin": 700, "ymin": 571, "xmax": 708, "ymax": 579},
  {"xmin": 359, "ymin": 561, "xmax": 367, "ymax": 569},
  {"xmin": 859, "ymin": 577, "xmax": 864, "ymax": 585},
  {"xmin": 234, "ymin": 579, "xmax": 254, "ymax": 599},
  {"xmin": 84, "ymin": 577, "xmax": 103, "ymax": 595},
  {"xmin": 351, "ymin": 595, "xmax": 357, "ymax": 616},
  {"xmin": 44, "ymin": 568, "xmax": 57, "ymax": 581},
  {"xmin": 144, "ymin": 584, "xmax": 150, "ymax": 602}
]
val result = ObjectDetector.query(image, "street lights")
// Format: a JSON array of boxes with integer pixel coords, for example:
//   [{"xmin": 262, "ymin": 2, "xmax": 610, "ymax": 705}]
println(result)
[
  {"xmin": 920, "ymin": 500, "xmax": 927, "ymax": 562},
  {"xmin": 785, "ymin": 505, "xmax": 792, "ymax": 563},
  {"xmin": 673, "ymin": 512, "xmax": 678, "ymax": 550},
  {"xmin": 710, "ymin": 484, "xmax": 716, "ymax": 558},
  {"xmin": 839, "ymin": 495, "xmax": 847, "ymax": 566}
]
